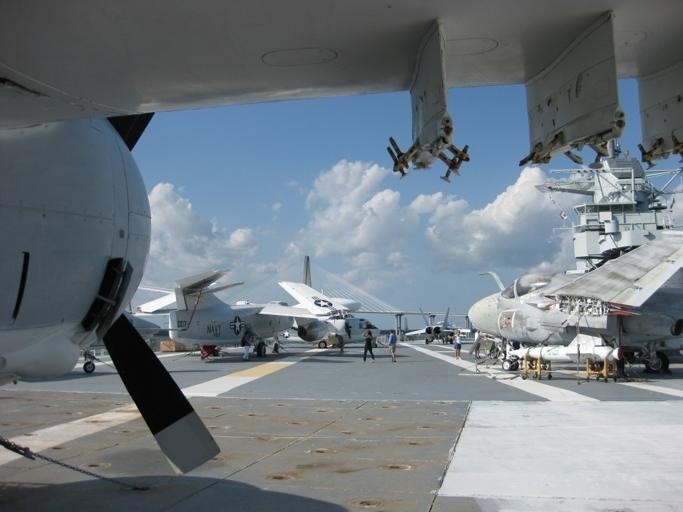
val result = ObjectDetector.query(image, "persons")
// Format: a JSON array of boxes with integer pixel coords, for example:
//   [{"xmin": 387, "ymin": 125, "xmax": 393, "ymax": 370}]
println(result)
[
  {"xmin": 241, "ymin": 331, "xmax": 252, "ymax": 361},
  {"xmin": 345, "ymin": 321, "xmax": 353, "ymax": 339},
  {"xmin": 255, "ymin": 340, "xmax": 268, "ymax": 361},
  {"xmin": 388, "ymin": 330, "xmax": 396, "ymax": 362},
  {"xmin": 453, "ymin": 330, "xmax": 461, "ymax": 359},
  {"xmin": 362, "ymin": 329, "xmax": 374, "ymax": 364},
  {"xmin": 335, "ymin": 333, "xmax": 344, "ymax": 352},
  {"xmin": 474, "ymin": 330, "xmax": 481, "ymax": 359}
]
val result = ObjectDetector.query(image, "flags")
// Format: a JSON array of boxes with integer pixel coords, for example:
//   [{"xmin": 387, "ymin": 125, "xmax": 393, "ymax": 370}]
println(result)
[{"xmin": 559, "ymin": 211, "xmax": 568, "ymax": 220}]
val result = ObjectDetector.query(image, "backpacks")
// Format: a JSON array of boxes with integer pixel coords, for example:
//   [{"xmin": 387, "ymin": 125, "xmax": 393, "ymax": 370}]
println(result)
[{"xmin": 241, "ymin": 336, "xmax": 248, "ymax": 347}]
